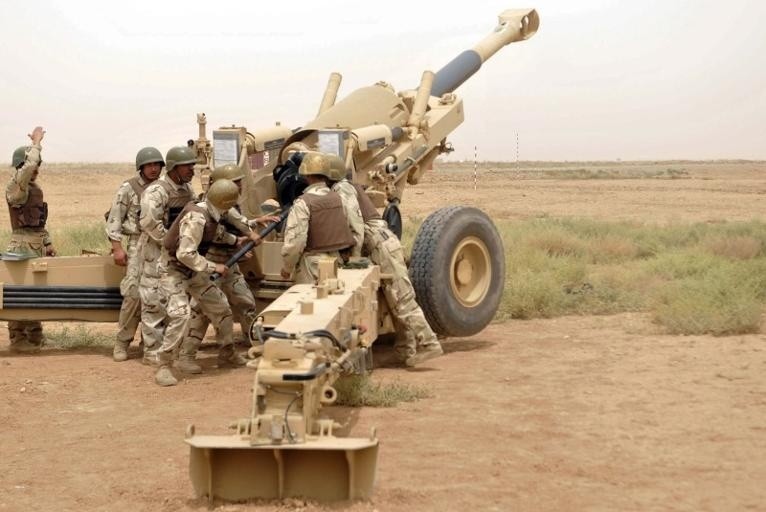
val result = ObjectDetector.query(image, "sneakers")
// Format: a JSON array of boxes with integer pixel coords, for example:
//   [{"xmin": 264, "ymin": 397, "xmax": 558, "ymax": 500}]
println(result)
[
  {"xmin": 217, "ymin": 356, "xmax": 247, "ymax": 369},
  {"xmin": 379, "ymin": 354, "xmax": 414, "ymax": 368},
  {"xmin": 142, "ymin": 355, "xmax": 160, "ymax": 365},
  {"xmin": 139, "ymin": 342, "xmax": 143, "ymax": 353},
  {"xmin": 178, "ymin": 358, "xmax": 201, "ymax": 373},
  {"xmin": 9, "ymin": 339, "xmax": 39, "ymax": 353},
  {"xmin": 27, "ymin": 330, "xmax": 54, "ymax": 347},
  {"xmin": 113, "ymin": 339, "xmax": 129, "ymax": 361},
  {"xmin": 407, "ymin": 348, "xmax": 443, "ymax": 366},
  {"xmin": 244, "ymin": 336, "xmax": 252, "ymax": 346},
  {"xmin": 154, "ymin": 367, "xmax": 177, "ymax": 386}
]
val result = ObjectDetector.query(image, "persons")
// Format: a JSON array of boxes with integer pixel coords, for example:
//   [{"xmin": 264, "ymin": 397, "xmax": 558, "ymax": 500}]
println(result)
[
  {"xmin": 279, "ymin": 149, "xmax": 356, "ymax": 286},
  {"xmin": 153, "ymin": 178, "xmax": 255, "ymax": 388},
  {"xmin": 174, "ymin": 164, "xmax": 282, "ymax": 375},
  {"xmin": 5, "ymin": 125, "xmax": 58, "ymax": 353},
  {"xmin": 324, "ymin": 153, "xmax": 444, "ymax": 367},
  {"xmin": 103, "ymin": 146, "xmax": 166, "ymax": 362},
  {"xmin": 137, "ymin": 146, "xmax": 197, "ymax": 368}
]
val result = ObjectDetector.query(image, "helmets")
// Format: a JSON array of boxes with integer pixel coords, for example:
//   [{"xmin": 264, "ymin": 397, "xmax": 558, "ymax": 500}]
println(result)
[
  {"xmin": 209, "ymin": 163, "xmax": 245, "ymax": 182},
  {"xmin": 327, "ymin": 153, "xmax": 347, "ymax": 180},
  {"xmin": 206, "ymin": 179, "xmax": 239, "ymax": 209},
  {"xmin": 135, "ymin": 147, "xmax": 165, "ymax": 172},
  {"xmin": 165, "ymin": 146, "xmax": 197, "ymax": 173},
  {"xmin": 10, "ymin": 146, "xmax": 42, "ymax": 168},
  {"xmin": 298, "ymin": 151, "xmax": 331, "ymax": 179}
]
